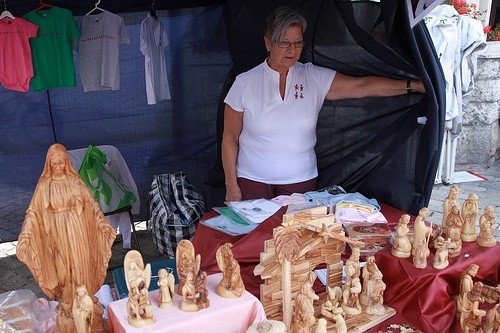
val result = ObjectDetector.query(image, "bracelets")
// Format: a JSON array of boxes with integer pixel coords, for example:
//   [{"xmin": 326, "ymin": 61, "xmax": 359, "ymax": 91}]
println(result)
[{"xmin": 406, "ymin": 79, "xmax": 412, "ymax": 93}]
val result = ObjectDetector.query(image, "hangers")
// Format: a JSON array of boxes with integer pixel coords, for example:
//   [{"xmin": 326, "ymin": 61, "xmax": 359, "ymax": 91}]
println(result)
[
  {"xmin": 35, "ymin": 0, "xmax": 51, "ymax": 10},
  {"xmin": 149, "ymin": 0, "xmax": 158, "ymax": 20},
  {"xmin": 86, "ymin": 0, "xmax": 105, "ymax": 16},
  {"xmin": 0, "ymin": 0, "xmax": 15, "ymax": 19}
]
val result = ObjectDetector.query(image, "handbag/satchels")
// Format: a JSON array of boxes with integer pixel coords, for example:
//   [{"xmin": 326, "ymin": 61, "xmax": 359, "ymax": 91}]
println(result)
[{"xmin": 146, "ymin": 171, "xmax": 206, "ymax": 257}]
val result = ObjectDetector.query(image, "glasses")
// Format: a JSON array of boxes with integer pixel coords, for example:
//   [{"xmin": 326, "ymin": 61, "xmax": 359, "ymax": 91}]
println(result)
[{"xmin": 275, "ymin": 38, "xmax": 305, "ymax": 48}]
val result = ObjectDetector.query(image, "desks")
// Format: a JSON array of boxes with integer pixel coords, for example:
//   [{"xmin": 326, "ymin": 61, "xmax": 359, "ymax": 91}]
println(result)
[{"xmin": 108, "ymin": 198, "xmax": 500, "ymax": 333}]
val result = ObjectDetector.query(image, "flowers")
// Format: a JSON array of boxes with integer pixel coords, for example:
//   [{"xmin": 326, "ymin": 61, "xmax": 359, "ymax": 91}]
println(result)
[
  {"xmin": 484, "ymin": 23, "xmax": 500, "ymax": 40},
  {"xmin": 452, "ymin": 0, "xmax": 487, "ymax": 20}
]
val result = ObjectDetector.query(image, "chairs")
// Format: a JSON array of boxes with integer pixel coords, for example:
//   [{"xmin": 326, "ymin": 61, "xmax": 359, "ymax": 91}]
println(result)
[{"xmin": 66, "ymin": 145, "xmax": 140, "ymax": 272}]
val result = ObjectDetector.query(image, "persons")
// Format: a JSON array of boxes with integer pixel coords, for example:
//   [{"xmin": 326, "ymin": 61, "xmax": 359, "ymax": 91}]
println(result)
[
  {"xmin": 221, "ymin": 9, "xmax": 426, "ymax": 201},
  {"xmin": 175, "ymin": 239, "xmax": 209, "ymax": 312},
  {"xmin": 124, "ymin": 250, "xmax": 156, "ymax": 327},
  {"xmin": 477, "ymin": 206, "xmax": 498, "ymax": 247},
  {"xmin": 391, "ymin": 214, "xmax": 412, "ymax": 258},
  {"xmin": 412, "ymin": 238, "xmax": 429, "ymax": 269},
  {"xmin": 456, "ymin": 264, "xmax": 500, "ymax": 333},
  {"xmin": 412, "ymin": 206, "xmax": 432, "ymax": 256},
  {"xmin": 16, "ymin": 143, "xmax": 116, "ymax": 333},
  {"xmin": 442, "ymin": 185, "xmax": 461, "ymax": 235},
  {"xmin": 157, "ymin": 269, "xmax": 175, "ymax": 309},
  {"xmin": 215, "ymin": 243, "xmax": 245, "ymax": 298},
  {"xmin": 433, "ymin": 236, "xmax": 449, "ymax": 270},
  {"xmin": 461, "ymin": 194, "xmax": 478, "ymax": 243},
  {"xmin": 292, "ymin": 248, "xmax": 386, "ymax": 333},
  {"xmin": 444, "ymin": 205, "xmax": 463, "ymax": 258}
]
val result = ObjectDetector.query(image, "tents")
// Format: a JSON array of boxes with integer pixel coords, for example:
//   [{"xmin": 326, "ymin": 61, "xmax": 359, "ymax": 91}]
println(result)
[
  {"xmin": 0, "ymin": 0, "xmax": 449, "ymax": 222},
  {"xmin": 422, "ymin": 5, "xmax": 487, "ymax": 185}
]
